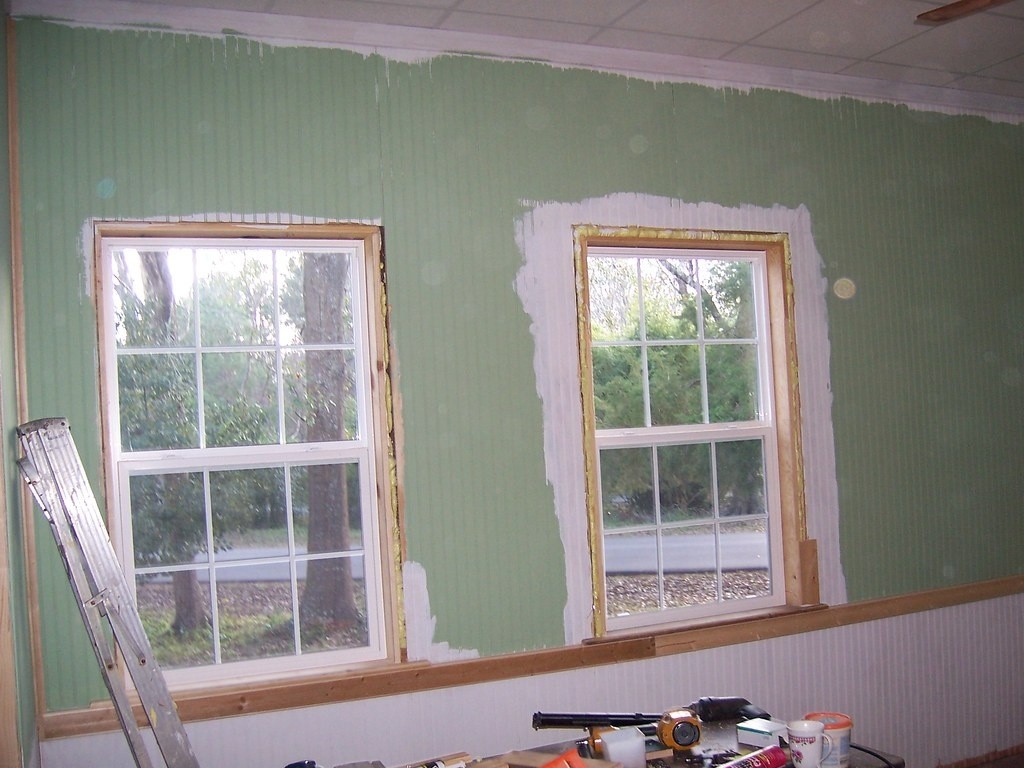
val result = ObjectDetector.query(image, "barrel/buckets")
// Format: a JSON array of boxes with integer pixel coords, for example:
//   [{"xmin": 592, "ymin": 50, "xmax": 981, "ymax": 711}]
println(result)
[{"xmin": 800, "ymin": 712, "xmax": 853, "ymax": 768}]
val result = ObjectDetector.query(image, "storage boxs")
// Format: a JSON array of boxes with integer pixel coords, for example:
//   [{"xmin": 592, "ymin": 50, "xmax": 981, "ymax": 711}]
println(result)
[{"xmin": 735, "ymin": 718, "xmax": 789, "ymax": 748}]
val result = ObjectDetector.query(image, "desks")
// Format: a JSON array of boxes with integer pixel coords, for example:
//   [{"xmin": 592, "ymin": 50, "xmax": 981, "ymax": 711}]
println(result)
[{"xmin": 470, "ymin": 717, "xmax": 905, "ymax": 768}]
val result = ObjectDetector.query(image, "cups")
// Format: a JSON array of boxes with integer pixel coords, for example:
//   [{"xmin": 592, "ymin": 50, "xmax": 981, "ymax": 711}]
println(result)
[{"xmin": 785, "ymin": 720, "xmax": 832, "ymax": 768}]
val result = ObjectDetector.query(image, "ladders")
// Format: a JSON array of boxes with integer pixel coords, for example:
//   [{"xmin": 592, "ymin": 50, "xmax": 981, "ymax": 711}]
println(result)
[{"xmin": 13, "ymin": 416, "xmax": 201, "ymax": 768}]
